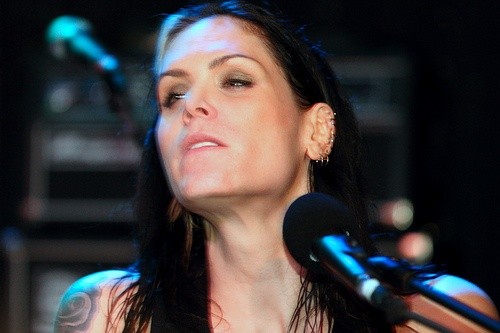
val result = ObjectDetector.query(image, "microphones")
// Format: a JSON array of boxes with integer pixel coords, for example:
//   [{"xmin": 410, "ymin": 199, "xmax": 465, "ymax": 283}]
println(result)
[
  {"xmin": 46, "ymin": 17, "xmax": 128, "ymax": 89},
  {"xmin": 281, "ymin": 193, "xmax": 411, "ymax": 326}
]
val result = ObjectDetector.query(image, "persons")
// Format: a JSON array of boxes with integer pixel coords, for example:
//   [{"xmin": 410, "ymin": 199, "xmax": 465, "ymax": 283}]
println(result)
[{"xmin": 49, "ymin": 0, "xmax": 500, "ymax": 333}]
